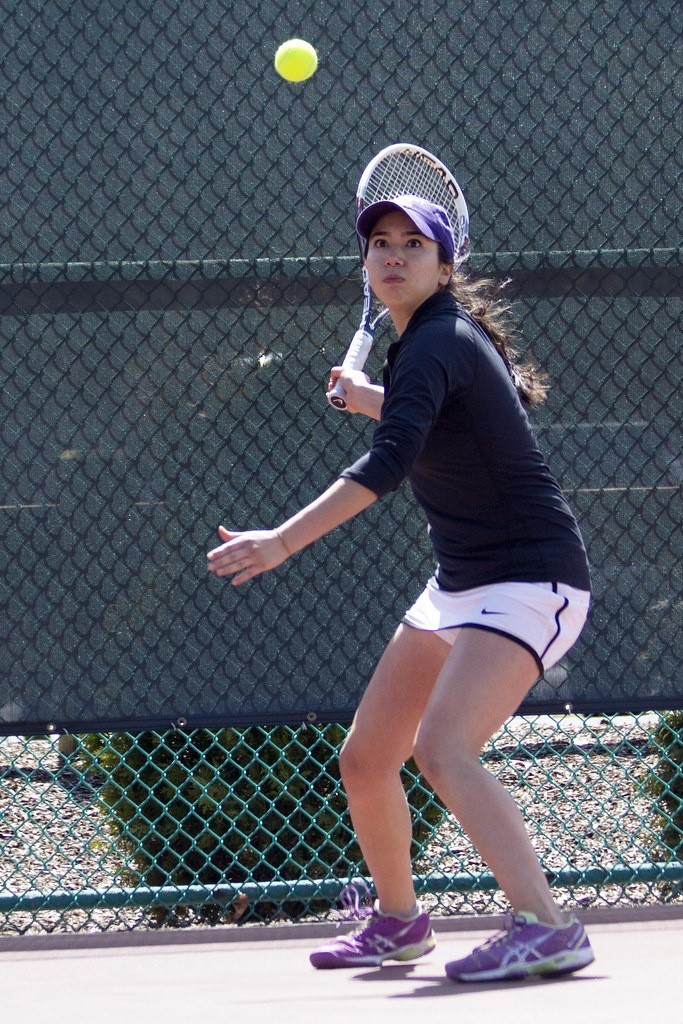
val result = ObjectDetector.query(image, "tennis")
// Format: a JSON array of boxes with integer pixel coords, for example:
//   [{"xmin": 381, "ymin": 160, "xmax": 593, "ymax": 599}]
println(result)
[{"xmin": 274, "ymin": 37, "xmax": 319, "ymax": 83}]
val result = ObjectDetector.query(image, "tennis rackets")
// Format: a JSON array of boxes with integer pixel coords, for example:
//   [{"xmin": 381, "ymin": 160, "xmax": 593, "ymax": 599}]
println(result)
[{"xmin": 327, "ymin": 141, "xmax": 472, "ymax": 412}]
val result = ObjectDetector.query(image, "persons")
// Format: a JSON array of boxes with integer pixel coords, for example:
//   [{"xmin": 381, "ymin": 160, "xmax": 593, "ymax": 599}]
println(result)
[{"xmin": 207, "ymin": 195, "xmax": 596, "ymax": 982}]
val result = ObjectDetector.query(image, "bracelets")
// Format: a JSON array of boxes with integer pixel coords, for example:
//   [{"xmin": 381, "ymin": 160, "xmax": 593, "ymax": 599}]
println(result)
[{"xmin": 274, "ymin": 528, "xmax": 291, "ymax": 556}]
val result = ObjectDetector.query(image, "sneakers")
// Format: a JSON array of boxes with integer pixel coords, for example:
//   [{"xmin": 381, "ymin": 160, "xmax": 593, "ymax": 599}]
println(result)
[
  {"xmin": 311, "ymin": 899, "xmax": 437, "ymax": 968},
  {"xmin": 446, "ymin": 913, "xmax": 596, "ymax": 982}
]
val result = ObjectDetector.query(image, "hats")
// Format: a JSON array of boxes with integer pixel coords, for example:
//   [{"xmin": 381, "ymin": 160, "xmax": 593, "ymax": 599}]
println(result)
[{"xmin": 355, "ymin": 196, "xmax": 455, "ymax": 260}]
